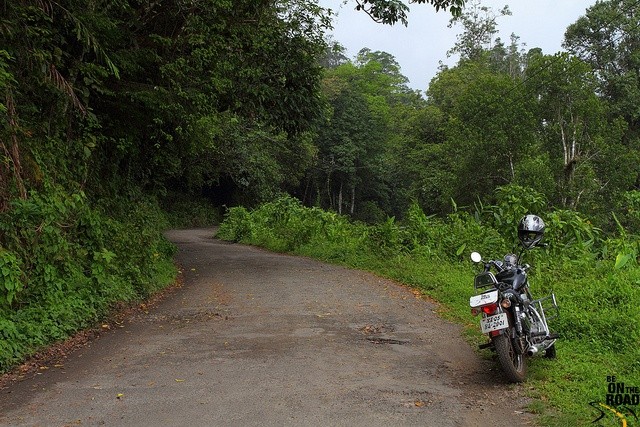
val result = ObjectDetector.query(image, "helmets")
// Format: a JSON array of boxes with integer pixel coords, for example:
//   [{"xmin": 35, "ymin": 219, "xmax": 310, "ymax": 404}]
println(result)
[{"xmin": 518, "ymin": 213, "xmax": 544, "ymax": 249}]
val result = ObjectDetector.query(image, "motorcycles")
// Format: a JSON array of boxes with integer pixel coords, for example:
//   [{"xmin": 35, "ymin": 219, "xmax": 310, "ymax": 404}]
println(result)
[{"xmin": 470, "ymin": 243, "xmax": 562, "ymax": 381}]
